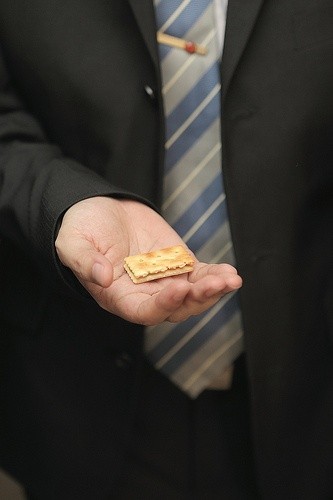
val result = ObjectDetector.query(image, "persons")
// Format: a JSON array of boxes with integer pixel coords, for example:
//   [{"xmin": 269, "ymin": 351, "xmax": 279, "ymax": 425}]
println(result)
[{"xmin": 0, "ymin": 0, "xmax": 332, "ymax": 499}]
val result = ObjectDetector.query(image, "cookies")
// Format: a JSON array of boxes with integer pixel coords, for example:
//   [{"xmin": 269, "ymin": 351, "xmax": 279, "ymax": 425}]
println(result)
[{"xmin": 122, "ymin": 245, "xmax": 195, "ymax": 284}]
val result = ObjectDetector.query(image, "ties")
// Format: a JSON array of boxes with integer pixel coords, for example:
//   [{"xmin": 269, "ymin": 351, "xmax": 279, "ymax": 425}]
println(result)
[{"xmin": 144, "ymin": 1, "xmax": 246, "ymax": 398}]
